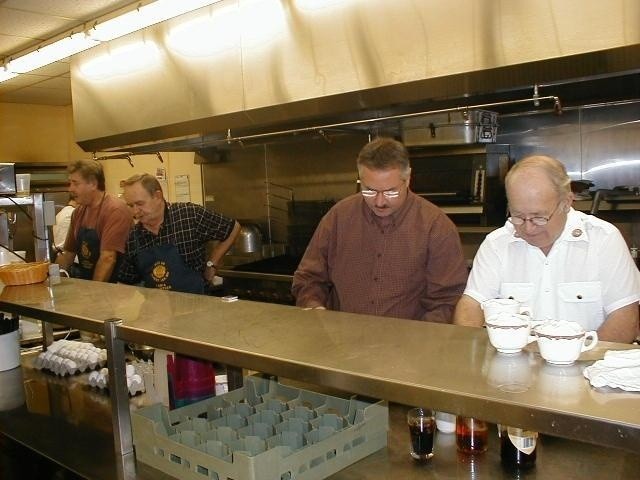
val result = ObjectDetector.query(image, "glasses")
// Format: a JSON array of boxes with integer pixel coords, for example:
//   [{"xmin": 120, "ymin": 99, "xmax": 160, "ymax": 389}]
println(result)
[
  {"xmin": 359, "ymin": 178, "xmax": 406, "ymax": 199},
  {"xmin": 505, "ymin": 199, "xmax": 563, "ymax": 226}
]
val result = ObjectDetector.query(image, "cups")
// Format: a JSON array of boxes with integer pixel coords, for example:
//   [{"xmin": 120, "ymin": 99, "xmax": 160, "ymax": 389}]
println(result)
[
  {"xmin": 485, "ymin": 315, "xmax": 539, "ymax": 354},
  {"xmin": 533, "ymin": 321, "xmax": 598, "ymax": 365},
  {"xmin": 499, "ymin": 423, "xmax": 538, "ymax": 470},
  {"xmin": 455, "ymin": 414, "xmax": 489, "ymax": 453},
  {"xmin": 15, "ymin": 174, "xmax": 31, "ymax": 197},
  {"xmin": 480, "ymin": 299, "xmax": 533, "ymax": 323},
  {"xmin": 407, "ymin": 407, "xmax": 436, "ymax": 460}
]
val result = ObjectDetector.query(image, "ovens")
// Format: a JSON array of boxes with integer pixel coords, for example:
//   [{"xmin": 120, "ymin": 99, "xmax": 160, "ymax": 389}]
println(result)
[{"xmin": 408, "ymin": 144, "xmax": 516, "ymax": 226}]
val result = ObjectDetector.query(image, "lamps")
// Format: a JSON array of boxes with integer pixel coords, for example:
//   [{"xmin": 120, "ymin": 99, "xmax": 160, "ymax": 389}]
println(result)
[
  {"xmin": 87, "ymin": 0, "xmax": 231, "ymax": 44},
  {"xmin": 0, "ymin": 24, "xmax": 104, "ymax": 84}
]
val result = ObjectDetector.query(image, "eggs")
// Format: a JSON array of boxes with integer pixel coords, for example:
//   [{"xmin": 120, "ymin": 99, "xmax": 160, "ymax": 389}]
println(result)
[
  {"xmin": 37, "ymin": 338, "xmax": 107, "ymax": 368},
  {"xmin": 88, "ymin": 368, "xmax": 108, "ymax": 384},
  {"xmin": 126, "ymin": 365, "xmax": 142, "ymax": 387}
]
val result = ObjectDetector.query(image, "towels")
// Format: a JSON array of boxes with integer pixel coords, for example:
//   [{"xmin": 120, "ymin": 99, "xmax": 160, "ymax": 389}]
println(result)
[{"xmin": 584, "ymin": 345, "xmax": 640, "ymax": 394}]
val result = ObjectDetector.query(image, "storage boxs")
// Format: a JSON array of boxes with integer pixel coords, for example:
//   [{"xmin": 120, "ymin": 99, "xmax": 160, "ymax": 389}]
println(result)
[
  {"xmin": 69, "ymin": 359, "xmax": 153, "ymax": 433},
  {"xmin": 20, "ymin": 347, "xmax": 135, "ymax": 427}
]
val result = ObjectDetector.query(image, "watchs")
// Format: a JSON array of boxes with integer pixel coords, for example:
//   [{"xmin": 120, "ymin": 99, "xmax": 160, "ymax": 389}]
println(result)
[{"xmin": 205, "ymin": 259, "xmax": 218, "ymax": 270}]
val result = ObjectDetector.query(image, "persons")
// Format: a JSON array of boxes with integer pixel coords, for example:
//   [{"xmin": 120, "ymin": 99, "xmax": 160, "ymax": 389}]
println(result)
[
  {"xmin": 452, "ymin": 155, "xmax": 640, "ymax": 345},
  {"xmin": 51, "ymin": 193, "xmax": 80, "ymax": 279},
  {"xmin": 115, "ymin": 172, "xmax": 242, "ymax": 295},
  {"xmin": 292, "ymin": 136, "xmax": 467, "ymax": 324},
  {"xmin": 56, "ymin": 159, "xmax": 135, "ymax": 283}
]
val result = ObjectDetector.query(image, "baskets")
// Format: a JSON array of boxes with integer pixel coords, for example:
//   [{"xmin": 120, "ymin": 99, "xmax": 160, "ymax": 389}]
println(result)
[{"xmin": 0, "ymin": 260, "xmax": 51, "ymax": 286}]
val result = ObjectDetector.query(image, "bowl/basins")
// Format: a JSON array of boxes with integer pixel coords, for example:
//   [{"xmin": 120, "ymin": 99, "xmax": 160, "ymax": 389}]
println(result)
[{"xmin": 129, "ymin": 343, "xmax": 155, "ymax": 359}]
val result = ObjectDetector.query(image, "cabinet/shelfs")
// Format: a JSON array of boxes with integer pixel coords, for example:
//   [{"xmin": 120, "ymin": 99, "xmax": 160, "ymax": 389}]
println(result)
[
  {"xmin": 0, "ymin": 276, "xmax": 640, "ymax": 478},
  {"xmin": 99, "ymin": 143, "xmax": 271, "ymax": 258}
]
